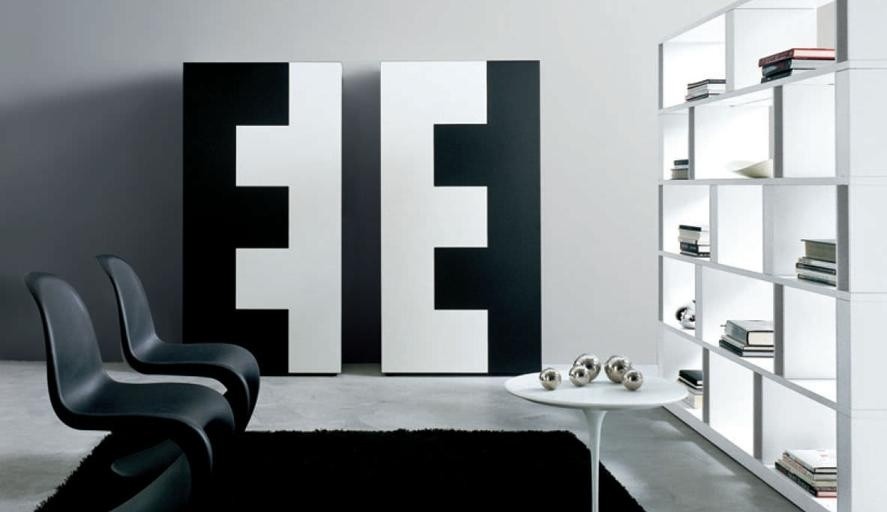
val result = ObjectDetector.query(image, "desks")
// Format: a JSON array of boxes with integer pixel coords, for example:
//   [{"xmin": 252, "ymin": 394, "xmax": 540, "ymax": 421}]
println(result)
[{"xmin": 503, "ymin": 368, "xmax": 689, "ymax": 512}]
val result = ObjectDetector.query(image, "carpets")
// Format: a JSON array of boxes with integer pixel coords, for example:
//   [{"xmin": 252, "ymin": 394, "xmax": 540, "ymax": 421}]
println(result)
[{"xmin": 34, "ymin": 429, "xmax": 649, "ymax": 512}]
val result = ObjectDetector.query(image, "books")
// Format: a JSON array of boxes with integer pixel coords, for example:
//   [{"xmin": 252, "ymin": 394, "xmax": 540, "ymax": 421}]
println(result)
[
  {"xmin": 677, "ymin": 223, "xmax": 709, "ymax": 260},
  {"xmin": 719, "ymin": 319, "xmax": 774, "ymax": 359},
  {"xmin": 684, "ymin": 77, "xmax": 726, "ymax": 104},
  {"xmin": 676, "ymin": 368, "xmax": 702, "ymax": 410},
  {"xmin": 757, "ymin": 47, "xmax": 837, "ymax": 83},
  {"xmin": 794, "ymin": 238, "xmax": 838, "ymax": 288},
  {"xmin": 670, "ymin": 159, "xmax": 688, "ymax": 182},
  {"xmin": 774, "ymin": 446, "xmax": 838, "ymax": 498}
]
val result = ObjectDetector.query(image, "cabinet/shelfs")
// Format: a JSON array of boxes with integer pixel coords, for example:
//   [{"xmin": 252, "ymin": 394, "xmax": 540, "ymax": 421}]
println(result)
[
  {"xmin": 380, "ymin": 60, "xmax": 543, "ymax": 377},
  {"xmin": 652, "ymin": 1, "xmax": 887, "ymax": 512},
  {"xmin": 181, "ymin": 61, "xmax": 345, "ymax": 376}
]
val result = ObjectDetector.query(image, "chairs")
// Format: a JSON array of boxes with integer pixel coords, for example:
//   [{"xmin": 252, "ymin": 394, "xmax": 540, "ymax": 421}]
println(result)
[
  {"xmin": 24, "ymin": 271, "xmax": 239, "ymax": 511},
  {"xmin": 97, "ymin": 255, "xmax": 261, "ymax": 479}
]
